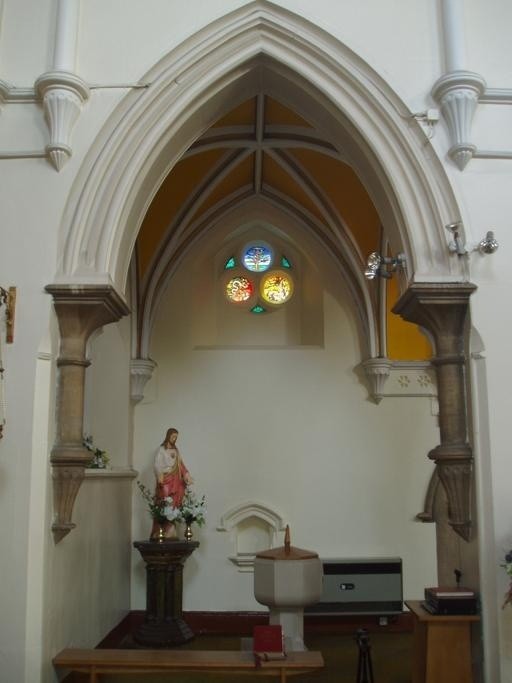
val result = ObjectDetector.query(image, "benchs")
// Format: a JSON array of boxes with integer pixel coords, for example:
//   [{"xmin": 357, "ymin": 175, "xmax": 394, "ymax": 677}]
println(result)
[{"xmin": 53, "ymin": 648, "xmax": 324, "ymax": 683}]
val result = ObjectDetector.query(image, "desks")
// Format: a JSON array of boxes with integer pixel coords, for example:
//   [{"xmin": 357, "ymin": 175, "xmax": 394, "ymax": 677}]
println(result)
[{"xmin": 402, "ymin": 600, "xmax": 481, "ymax": 683}]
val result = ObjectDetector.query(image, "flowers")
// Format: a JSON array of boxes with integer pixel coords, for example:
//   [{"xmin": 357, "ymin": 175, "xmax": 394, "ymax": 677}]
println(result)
[
  {"xmin": 80, "ymin": 432, "xmax": 111, "ymax": 468},
  {"xmin": 137, "ymin": 481, "xmax": 209, "ymax": 528}
]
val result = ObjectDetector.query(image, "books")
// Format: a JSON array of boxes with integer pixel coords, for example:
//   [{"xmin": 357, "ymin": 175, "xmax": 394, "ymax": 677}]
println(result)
[
  {"xmin": 429, "ymin": 584, "xmax": 475, "ymax": 598},
  {"xmin": 253, "ymin": 624, "xmax": 284, "ymax": 652}
]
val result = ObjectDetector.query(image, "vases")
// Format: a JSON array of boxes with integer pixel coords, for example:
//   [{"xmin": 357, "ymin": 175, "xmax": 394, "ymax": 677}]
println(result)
[
  {"xmin": 185, "ymin": 525, "xmax": 193, "ymax": 541},
  {"xmin": 153, "ymin": 525, "xmax": 166, "ymax": 542}
]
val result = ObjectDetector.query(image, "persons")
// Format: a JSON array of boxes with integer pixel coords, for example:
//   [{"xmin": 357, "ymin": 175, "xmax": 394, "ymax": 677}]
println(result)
[{"xmin": 150, "ymin": 428, "xmax": 194, "ymax": 542}]
